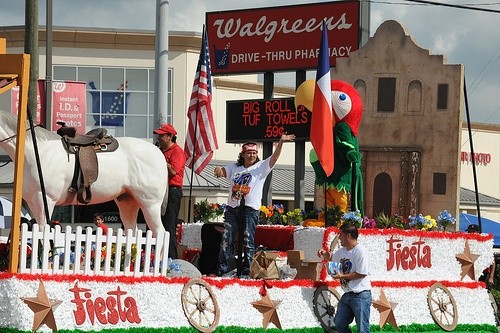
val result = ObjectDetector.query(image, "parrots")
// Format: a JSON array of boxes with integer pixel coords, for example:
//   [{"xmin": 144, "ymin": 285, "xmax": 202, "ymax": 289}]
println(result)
[{"xmin": 295, "ymin": 79, "xmax": 364, "ymax": 228}]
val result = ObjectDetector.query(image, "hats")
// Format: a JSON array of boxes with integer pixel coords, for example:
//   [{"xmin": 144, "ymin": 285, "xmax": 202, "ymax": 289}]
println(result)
[
  {"xmin": 465, "ymin": 224, "xmax": 480, "ymax": 232},
  {"xmin": 153, "ymin": 124, "xmax": 177, "ymax": 135}
]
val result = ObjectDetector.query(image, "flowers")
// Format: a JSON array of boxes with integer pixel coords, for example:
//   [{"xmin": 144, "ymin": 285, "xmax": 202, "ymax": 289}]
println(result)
[
  {"xmin": 192, "ymin": 198, "xmax": 458, "ymax": 229},
  {"xmin": 21, "ymin": 242, "xmax": 181, "ymax": 274}
]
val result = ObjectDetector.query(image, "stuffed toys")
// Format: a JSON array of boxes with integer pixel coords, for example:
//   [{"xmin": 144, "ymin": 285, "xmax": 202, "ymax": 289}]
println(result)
[{"xmin": 294, "ymin": 80, "xmax": 363, "ymax": 228}]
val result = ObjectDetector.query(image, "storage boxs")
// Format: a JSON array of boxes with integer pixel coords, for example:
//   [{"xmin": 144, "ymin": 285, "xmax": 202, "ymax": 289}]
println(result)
[
  {"xmin": 287, "ymin": 257, "xmax": 324, "ymax": 279},
  {"xmin": 288, "ymin": 249, "xmax": 306, "ymax": 260}
]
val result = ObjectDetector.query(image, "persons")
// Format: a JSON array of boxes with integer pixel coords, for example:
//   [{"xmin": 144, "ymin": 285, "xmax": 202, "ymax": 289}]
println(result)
[
  {"xmin": 90, "ymin": 213, "xmax": 109, "ymax": 250},
  {"xmin": 215, "ymin": 132, "xmax": 296, "ymax": 279},
  {"xmin": 153, "ymin": 124, "xmax": 186, "ymax": 259},
  {"xmin": 317, "ymin": 223, "xmax": 372, "ymax": 333},
  {"xmin": 465, "ymin": 224, "xmax": 495, "ymax": 286}
]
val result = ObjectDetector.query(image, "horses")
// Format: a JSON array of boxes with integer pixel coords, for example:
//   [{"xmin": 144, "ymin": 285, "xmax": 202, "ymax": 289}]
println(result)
[{"xmin": 0, "ymin": 109, "xmax": 168, "ymax": 261}]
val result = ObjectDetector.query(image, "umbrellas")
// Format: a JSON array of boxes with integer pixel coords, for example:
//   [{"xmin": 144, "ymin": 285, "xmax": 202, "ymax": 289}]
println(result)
[{"xmin": 459, "ymin": 213, "xmax": 500, "ymax": 247}]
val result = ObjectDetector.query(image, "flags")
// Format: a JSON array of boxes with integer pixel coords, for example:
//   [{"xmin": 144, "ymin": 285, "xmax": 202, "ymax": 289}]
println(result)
[
  {"xmin": 184, "ymin": 27, "xmax": 219, "ymax": 175},
  {"xmin": 310, "ymin": 21, "xmax": 334, "ymax": 177}
]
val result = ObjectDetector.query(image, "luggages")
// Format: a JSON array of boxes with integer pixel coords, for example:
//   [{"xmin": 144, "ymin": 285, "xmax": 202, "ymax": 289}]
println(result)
[{"xmin": 198, "ymin": 222, "xmax": 233, "ymax": 276}]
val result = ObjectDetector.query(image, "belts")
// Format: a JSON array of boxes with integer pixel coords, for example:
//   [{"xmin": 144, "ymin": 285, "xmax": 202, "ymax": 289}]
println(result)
[{"xmin": 170, "ymin": 186, "xmax": 179, "ymax": 190}]
